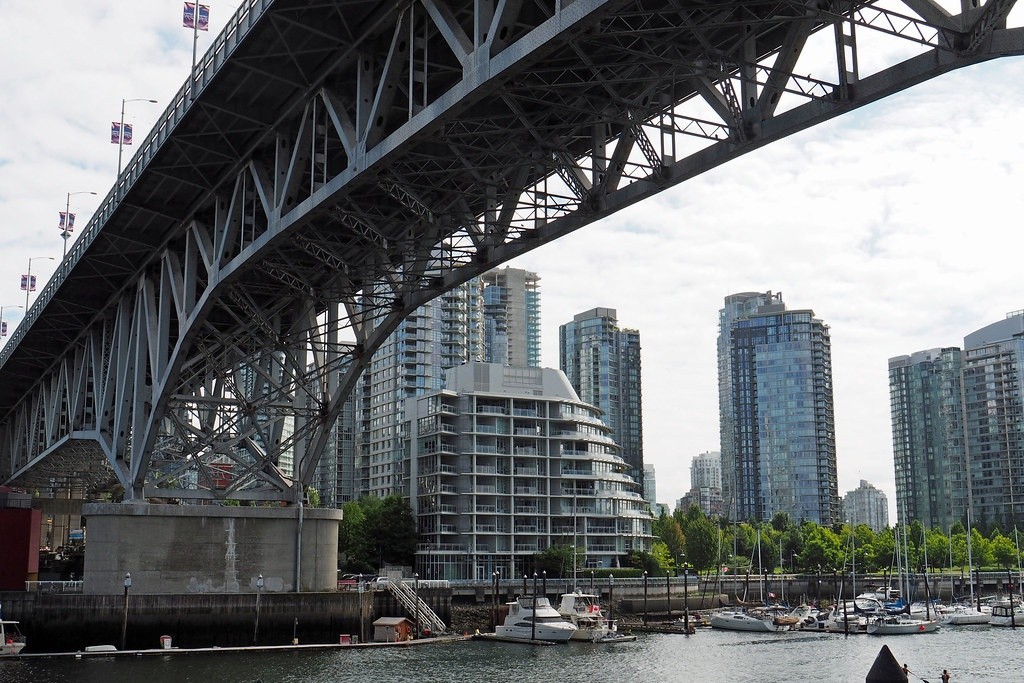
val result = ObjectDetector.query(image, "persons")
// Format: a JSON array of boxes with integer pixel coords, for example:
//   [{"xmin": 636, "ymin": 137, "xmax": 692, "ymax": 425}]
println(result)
[
  {"xmin": 903, "ymin": 664, "xmax": 910, "ymax": 676},
  {"xmin": 942, "ymin": 670, "xmax": 949, "ymax": 683}
]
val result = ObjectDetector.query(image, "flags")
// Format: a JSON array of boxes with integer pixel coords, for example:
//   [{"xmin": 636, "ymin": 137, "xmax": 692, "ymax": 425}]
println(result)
[
  {"xmin": 769, "ymin": 593, "xmax": 776, "ymax": 597},
  {"xmin": 589, "ymin": 605, "xmax": 600, "ymax": 614}
]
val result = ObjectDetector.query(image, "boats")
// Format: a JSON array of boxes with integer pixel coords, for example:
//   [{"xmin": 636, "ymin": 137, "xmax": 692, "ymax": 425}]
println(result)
[
  {"xmin": 711, "ymin": 609, "xmax": 791, "ymax": 632},
  {"xmin": 555, "ymin": 592, "xmax": 637, "ymax": 641},
  {"xmin": 867, "ymin": 617, "xmax": 940, "ymax": 634},
  {"xmin": 989, "ymin": 604, "xmax": 1024, "ymax": 628},
  {"xmin": 492, "ymin": 594, "xmax": 578, "ymax": 642},
  {"xmin": 942, "ymin": 608, "xmax": 990, "ymax": 625},
  {"xmin": 671, "ymin": 586, "xmax": 1024, "ymax": 635}
]
